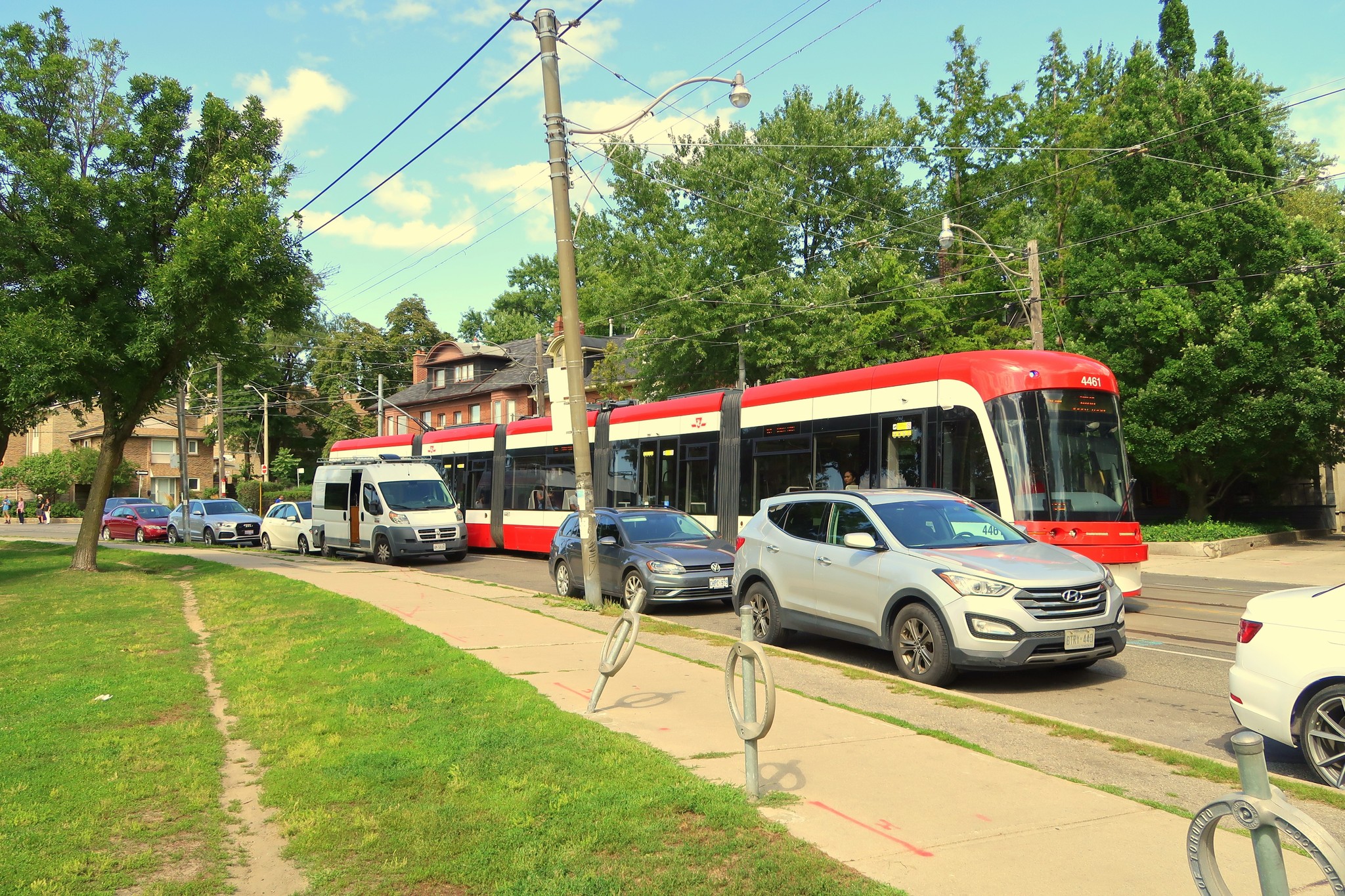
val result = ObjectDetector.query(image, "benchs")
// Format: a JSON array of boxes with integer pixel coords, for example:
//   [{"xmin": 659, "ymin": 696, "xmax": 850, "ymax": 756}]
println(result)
[
  {"xmin": 562, "ymin": 490, "xmax": 576, "ymax": 510},
  {"xmin": 528, "ymin": 490, "xmax": 564, "ymax": 510}
]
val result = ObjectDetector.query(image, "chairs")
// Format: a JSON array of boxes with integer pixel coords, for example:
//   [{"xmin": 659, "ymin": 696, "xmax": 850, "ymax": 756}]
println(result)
[
  {"xmin": 139, "ymin": 509, "xmax": 147, "ymax": 518},
  {"xmin": 382, "ymin": 486, "xmax": 396, "ymax": 505}
]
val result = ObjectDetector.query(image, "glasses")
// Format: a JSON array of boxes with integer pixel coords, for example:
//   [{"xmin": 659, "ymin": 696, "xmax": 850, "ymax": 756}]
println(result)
[{"xmin": 1030, "ymin": 470, "xmax": 1037, "ymax": 474}]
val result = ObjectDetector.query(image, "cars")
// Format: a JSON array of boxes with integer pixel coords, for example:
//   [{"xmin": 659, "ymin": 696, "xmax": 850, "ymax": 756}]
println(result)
[
  {"xmin": 1228, "ymin": 580, "xmax": 1345, "ymax": 789},
  {"xmin": 260, "ymin": 500, "xmax": 322, "ymax": 555},
  {"xmin": 100, "ymin": 503, "xmax": 174, "ymax": 543},
  {"xmin": 166, "ymin": 498, "xmax": 263, "ymax": 547},
  {"xmin": 103, "ymin": 497, "xmax": 155, "ymax": 516}
]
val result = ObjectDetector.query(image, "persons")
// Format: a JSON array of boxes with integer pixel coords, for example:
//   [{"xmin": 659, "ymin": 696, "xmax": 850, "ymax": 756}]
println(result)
[
  {"xmin": 36, "ymin": 494, "xmax": 45, "ymax": 524},
  {"xmin": 44, "ymin": 498, "xmax": 51, "ymax": 524},
  {"xmin": 275, "ymin": 495, "xmax": 284, "ymax": 504},
  {"xmin": 570, "ymin": 489, "xmax": 578, "ymax": 510},
  {"xmin": 536, "ymin": 482, "xmax": 560, "ymax": 510},
  {"xmin": 0, "ymin": 495, "xmax": 11, "ymax": 524},
  {"xmin": 1016, "ymin": 463, "xmax": 1045, "ymax": 495},
  {"xmin": 17, "ymin": 497, "xmax": 25, "ymax": 525},
  {"xmin": 844, "ymin": 471, "xmax": 857, "ymax": 485}
]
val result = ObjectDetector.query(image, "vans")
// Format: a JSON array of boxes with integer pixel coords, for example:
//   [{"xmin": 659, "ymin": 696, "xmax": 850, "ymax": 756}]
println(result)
[{"xmin": 312, "ymin": 453, "xmax": 468, "ymax": 566}]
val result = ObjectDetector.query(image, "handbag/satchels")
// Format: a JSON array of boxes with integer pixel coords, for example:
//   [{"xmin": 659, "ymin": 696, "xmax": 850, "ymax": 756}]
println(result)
[{"xmin": 18, "ymin": 509, "xmax": 21, "ymax": 512}]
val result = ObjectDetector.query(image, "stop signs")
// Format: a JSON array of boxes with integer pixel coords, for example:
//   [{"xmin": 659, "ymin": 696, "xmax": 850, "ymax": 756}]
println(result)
[{"xmin": 262, "ymin": 465, "xmax": 267, "ymax": 473}]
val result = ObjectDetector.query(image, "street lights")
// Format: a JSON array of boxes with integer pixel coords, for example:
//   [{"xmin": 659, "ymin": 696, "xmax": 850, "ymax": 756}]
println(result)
[
  {"xmin": 938, "ymin": 213, "xmax": 1045, "ymax": 351},
  {"xmin": 544, "ymin": 72, "xmax": 754, "ymax": 610},
  {"xmin": 472, "ymin": 333, "xmax": 545, "ymax": 419},
  {"xmin": 243, "ymin": 383, "xmax": 269, "ymax": 483}
]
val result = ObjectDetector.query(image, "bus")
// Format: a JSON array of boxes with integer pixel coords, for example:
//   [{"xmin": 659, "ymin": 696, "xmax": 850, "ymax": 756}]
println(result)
[{"xmin": 327, "ymin": 348, "xmax": 1151, "ymax": 614}]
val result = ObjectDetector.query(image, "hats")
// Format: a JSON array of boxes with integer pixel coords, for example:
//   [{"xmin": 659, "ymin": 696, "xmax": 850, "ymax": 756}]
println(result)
[{"xmin": 37, "ymin": 494, "xmax": 45, "ymax": 499}]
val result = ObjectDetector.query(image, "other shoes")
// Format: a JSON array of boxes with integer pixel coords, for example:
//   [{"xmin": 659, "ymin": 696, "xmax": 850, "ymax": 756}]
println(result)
[
  {"xmin": 8, "ymin": 522, "xmax": 10, "ymax": 524},
  {"xmin": 5, "ymin": 521, "xmax": 8, "ymax": 523},
  {"xmin": 38, "ymin": 522, "xmax": 44, "ymax": 524},
  {"xmin": 19, "ymin": 523, "xmax": 23, "ymax": 524},
  {"xmin": 46, "ymin": 523, "xmax": 50, "ymax": 524}
]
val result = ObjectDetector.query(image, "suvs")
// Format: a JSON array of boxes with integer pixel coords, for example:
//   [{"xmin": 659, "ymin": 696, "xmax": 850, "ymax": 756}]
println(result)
[
  {"xmin": 548, "ymin": 505, "xmax": 737, "ymax": 616},
  {"xmin": 731, "ymin": 488, "xmax": 1127, "ymax": 689}
]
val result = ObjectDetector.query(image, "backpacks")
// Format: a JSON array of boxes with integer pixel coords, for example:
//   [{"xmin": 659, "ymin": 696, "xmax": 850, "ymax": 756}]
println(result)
[
  {"xmin": 5, "ymin": 499, "xmax": 12, "ymax": 509},
  {"xmin": 36, "ymin": 498, "xmax": 43, "ymax": 508}
]
